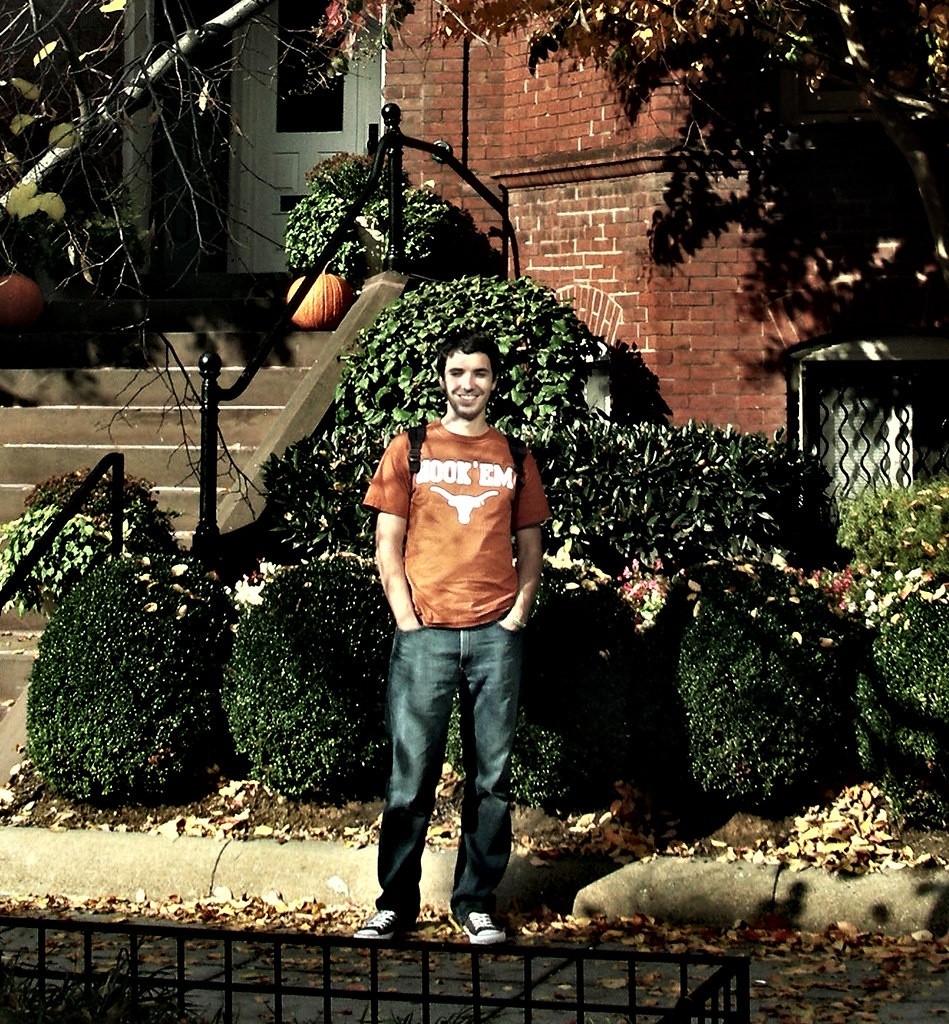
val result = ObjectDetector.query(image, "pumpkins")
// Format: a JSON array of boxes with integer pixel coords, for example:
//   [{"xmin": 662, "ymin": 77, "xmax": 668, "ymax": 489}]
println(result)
[
  {"xmin": 0, "ymin": 272, "xmax": 45, "ymax": 328},
  {"xmin": 287, "ymin": 263, "xmax": 352, "ymax": 331}
]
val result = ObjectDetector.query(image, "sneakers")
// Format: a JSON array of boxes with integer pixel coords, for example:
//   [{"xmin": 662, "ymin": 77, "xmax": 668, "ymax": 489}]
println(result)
[
  {"xmin": 353, "ymin": 910, "xmax": 418, "ymax": 941},
  {"xmin": 462, "ymin": 911, "xmax": 506, "ymax": 945}
]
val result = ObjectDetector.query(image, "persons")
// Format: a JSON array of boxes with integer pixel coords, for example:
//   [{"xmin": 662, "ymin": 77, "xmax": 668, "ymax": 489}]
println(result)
[{"xmin": 353, "ymin": 332, "xmax": 555, "ymax": 946}]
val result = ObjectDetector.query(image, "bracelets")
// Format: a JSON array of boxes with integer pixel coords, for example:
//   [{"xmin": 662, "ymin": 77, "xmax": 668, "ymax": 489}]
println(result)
[{"xmin": 514, "ymin": 619, "xmax": 526, "ymax": 629}]
